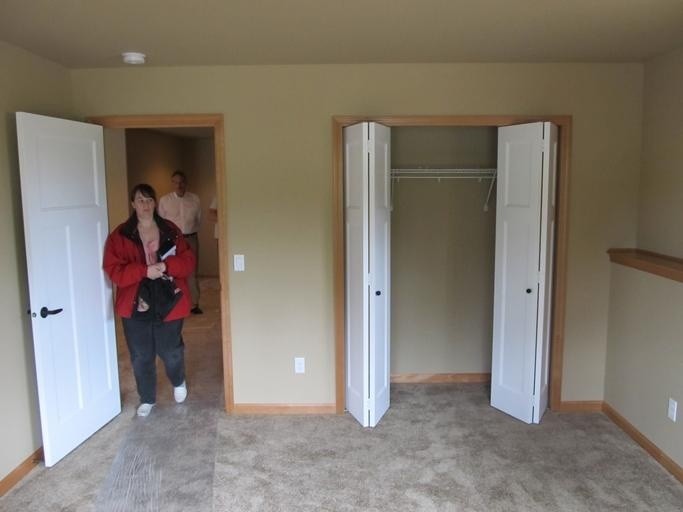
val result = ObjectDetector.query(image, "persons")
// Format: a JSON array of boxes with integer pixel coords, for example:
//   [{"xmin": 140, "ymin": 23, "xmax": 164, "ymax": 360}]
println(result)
[
  {"xmin": 158, "ymin": 171, "xmax": 202, "ymax": 315},
  {"xmin": 208, "ymin": 196, "xmax": 218, "ymax": 241},
  {"xmin": 104, "ymin": 183, "xmax": 196, "ymax": 418}
]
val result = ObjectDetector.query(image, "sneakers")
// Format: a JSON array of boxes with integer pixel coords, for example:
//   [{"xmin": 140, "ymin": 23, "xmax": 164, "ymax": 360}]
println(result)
[
  {"xmin": 137, "ymin": 402, "xmax": 156, "ymax": 416},
  {"xmin": 174, "ymin": 381, "xmax": 187, "ymax": 403}
]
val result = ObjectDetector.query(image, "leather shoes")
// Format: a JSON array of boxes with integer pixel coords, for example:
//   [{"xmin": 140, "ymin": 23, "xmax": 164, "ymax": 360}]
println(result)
[{"xmin": 191, "ymin": 307, "xmax": 202, "ymax": 314}]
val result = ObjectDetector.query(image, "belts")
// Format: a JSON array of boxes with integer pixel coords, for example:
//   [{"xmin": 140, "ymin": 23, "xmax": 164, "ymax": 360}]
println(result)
[{"xmin": 184, "ymin": 233, "xmax": 196, "ymax": 238}]
